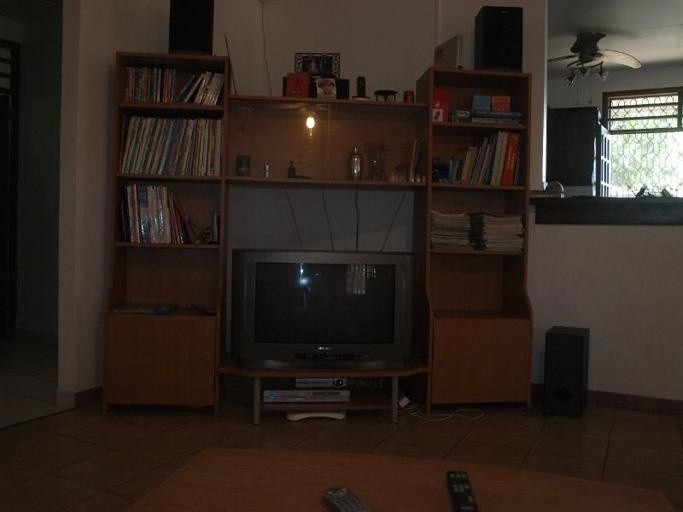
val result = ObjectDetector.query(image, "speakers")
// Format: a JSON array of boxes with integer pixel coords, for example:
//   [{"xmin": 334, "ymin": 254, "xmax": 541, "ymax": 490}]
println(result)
[
  {"xmin": 169, "ymin": 0, "xmax": 214, "ymax": 55},
  {"xmin": 544, "ymin": 327, "xmax": 589, "ymax": 417},
  {"xmin": 475, "ymin": 7, "xmax": 522, "ymax": 72}
]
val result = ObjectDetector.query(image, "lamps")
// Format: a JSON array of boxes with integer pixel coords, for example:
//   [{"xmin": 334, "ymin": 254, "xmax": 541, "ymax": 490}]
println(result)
[{"xmin": 560, "ymin": 50, "xmax": 609, "ymax": 89}]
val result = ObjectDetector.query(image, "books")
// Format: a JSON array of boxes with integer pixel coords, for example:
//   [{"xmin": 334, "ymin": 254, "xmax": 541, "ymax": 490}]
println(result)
[
  {"xmin": 431, "ymin": 112, "xmax": 526, "ymax": 252},
  {"xmin": 117, "ymin": 67, "xmax": 221, "ymax": 243}
]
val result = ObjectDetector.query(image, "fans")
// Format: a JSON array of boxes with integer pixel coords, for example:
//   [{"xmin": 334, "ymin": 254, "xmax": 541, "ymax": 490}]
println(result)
[{"xmin": 546, "ymin": 35, "xmax": 645, "ymax": 69}]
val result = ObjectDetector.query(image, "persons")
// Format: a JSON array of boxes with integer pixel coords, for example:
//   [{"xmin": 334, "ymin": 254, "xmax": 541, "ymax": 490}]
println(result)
[{"xmin": 317, "ymin": 79, "xmax": 335, "ymax": 96}]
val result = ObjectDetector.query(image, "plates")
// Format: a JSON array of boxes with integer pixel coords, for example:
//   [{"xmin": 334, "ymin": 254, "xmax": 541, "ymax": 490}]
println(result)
[{"xmin": 351, "ymin": 97, "xmax": 372, "ymax": 100}]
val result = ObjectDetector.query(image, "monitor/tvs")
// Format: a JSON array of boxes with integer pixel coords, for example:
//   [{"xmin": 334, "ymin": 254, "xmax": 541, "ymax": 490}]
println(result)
[{"xmin": 231, "ymin": 248, "xmax": 415, "ymax": 371}]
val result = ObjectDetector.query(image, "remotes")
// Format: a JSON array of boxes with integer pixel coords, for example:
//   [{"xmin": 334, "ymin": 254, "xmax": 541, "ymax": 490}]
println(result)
[
  {"xmin": 446, "ymin": 470, "xmax": 477, "ymax": 512},
  {"xmin": 323, "ymin": 486, "xmax": 367, "ymax": 512}
]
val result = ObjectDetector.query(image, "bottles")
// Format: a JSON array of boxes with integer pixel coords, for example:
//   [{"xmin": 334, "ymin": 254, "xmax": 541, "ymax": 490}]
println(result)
[
  {"xmin": 404, "ymin": 90, "xmax": 415, "ymax": 104},
  {"xmin": 347, "ymin": 145, "xmax": 362, "ymax": 180}
]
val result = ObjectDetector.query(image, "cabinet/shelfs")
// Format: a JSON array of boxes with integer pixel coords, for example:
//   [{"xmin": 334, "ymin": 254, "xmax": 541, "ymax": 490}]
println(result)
[{"xmin": 102, "ymin": 47, "xmax": 538, "ymax": 425}]
